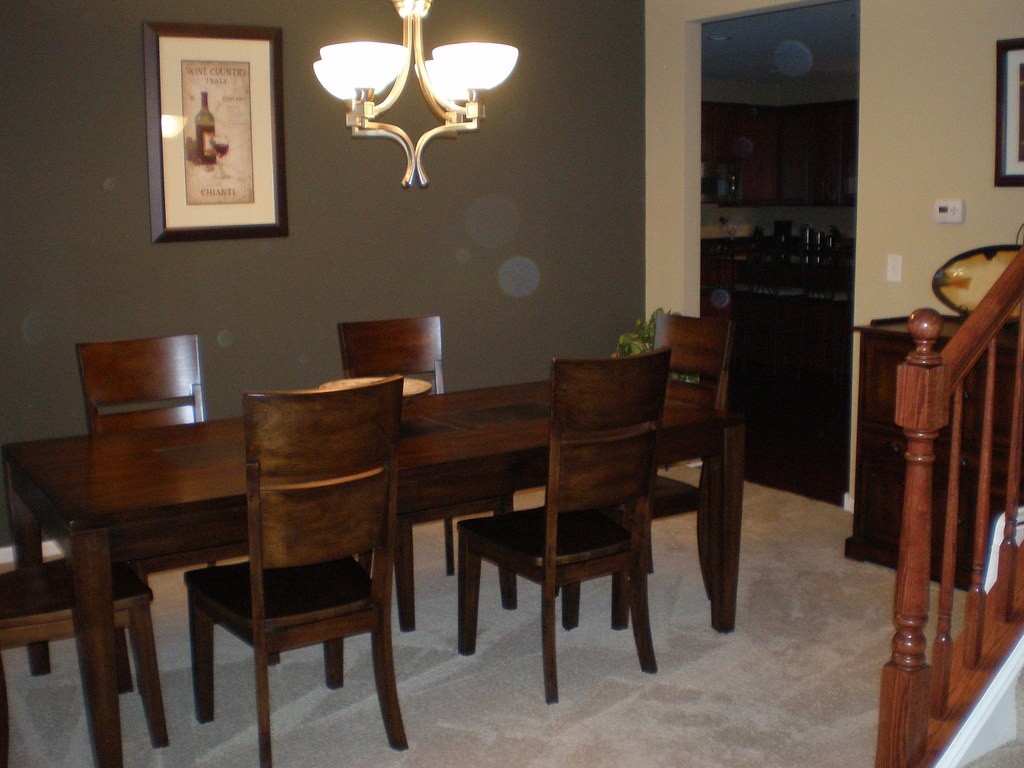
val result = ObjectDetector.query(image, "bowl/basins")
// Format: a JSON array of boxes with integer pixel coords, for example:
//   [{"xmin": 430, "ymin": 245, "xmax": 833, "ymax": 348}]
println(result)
[{"xmin": 317, "ymin": 376, "xmax": 432, "ymax": 414}]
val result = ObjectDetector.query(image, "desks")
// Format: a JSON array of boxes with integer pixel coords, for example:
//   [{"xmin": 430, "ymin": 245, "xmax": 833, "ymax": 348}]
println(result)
[{"xmin": 0, "ymin": 373, "xmax": 737, "ymax": 768}]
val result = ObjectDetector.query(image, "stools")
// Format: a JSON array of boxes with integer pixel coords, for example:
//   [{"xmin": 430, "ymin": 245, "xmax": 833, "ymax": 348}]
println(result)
[{"xmin": 0, "ymin": 558, "xmax": 170, "ymax": 768}]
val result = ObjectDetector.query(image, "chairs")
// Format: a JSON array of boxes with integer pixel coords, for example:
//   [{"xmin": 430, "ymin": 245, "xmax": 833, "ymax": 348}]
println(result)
[
  {"xmin": 641, "ymin": 315, "xmax": 739, "ymax": 600},
  {"xmin": 182, "ymin": 373, "xmax": 409, "ymax": 768},
  {"xmin": 455, "ymin": 343, "xmax": 672, "ymax": 704},
  {"xmin": 332, "ymin": 315, "xmax": 518, "ymax": 632}
]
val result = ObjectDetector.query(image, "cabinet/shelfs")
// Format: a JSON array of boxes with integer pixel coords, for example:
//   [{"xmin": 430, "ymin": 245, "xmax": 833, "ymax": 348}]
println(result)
[{"xmin": 845, "ymin": 313, "xmax": 1019, "ymax": 592}]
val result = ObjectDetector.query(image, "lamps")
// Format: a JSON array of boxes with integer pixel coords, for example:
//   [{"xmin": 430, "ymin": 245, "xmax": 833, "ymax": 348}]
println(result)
[{"xmin": 314, "ymin": 0, "xmax": 519, "ymax": 189}]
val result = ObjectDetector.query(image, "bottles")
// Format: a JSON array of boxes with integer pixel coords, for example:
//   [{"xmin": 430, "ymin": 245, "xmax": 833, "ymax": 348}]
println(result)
[
  {"xmin": 798, "ymin": 223, "xmax": 835, "ymax": 249},
  {"xmin": 753, "ymin": 226, "xmax": 763, "ymax": 245}
]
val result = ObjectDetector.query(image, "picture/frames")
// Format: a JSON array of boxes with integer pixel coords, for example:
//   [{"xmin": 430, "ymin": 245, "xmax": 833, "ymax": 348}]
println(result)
[
  {"xmin": 993, "ymin": 38, "xmax": 1024, "ymax": 187},
  {"xmin": 142, "ymin": 22, "xmax": 289, "ymax": 244}
]
